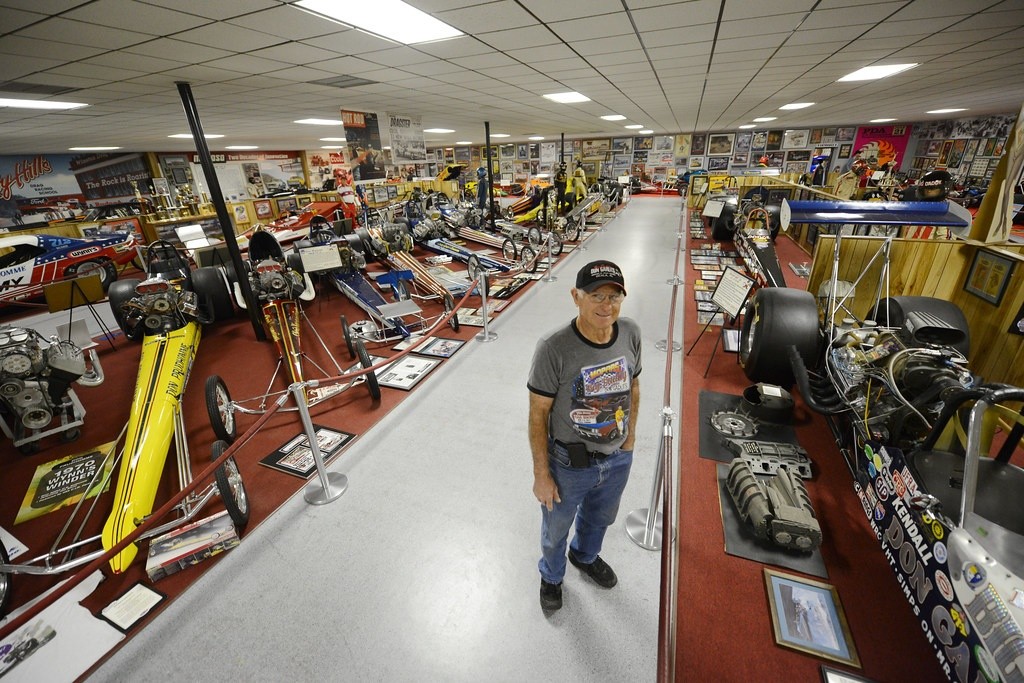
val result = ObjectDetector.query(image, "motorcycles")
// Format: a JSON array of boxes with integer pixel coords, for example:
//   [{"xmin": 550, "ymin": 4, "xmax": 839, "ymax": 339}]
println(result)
[
  {"xmin": 962, "ymin": 189, "xmax": 986, "ymax": 209},
  {"xmin": 0, "ymin": 176, "xmax": 641, "ymax": 618},
  {"xmin": 709, "ymin": 198, "xmax": 787, "ymax": 288},
  {"xmin": 744, "ymin": 287, "xmax": 1024, "ymax": 683},
  {"xmin": 677, "ymin": 170, "xmax": 707, "ymax": 196}
]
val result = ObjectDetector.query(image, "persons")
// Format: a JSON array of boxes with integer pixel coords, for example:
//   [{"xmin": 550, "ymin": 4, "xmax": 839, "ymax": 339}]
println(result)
[
  {"xmin": 407, "ymin": 167, "xmax": 415, "ymax": 181},
  {"xmin": 248, "ymin": 176, "xmax": 261, "ymax": 197},
  {"xmin": 336, "ymin": 168, "xmax": 359, "ymax": 230},
  {"xmin": 812, "ymin": 151, "xmax": 892, "ymax": 200},
  {"xmin": 527, "ymin": 260, "xmax": 642, "ymax": 610},
  {"xmin": 572, "ymin": 161, "xmax": 588, "ymax": 207},
  {"xmin": 755, "ymin": 157, "xmax": 767, "ymax": 169},
  {"xmin": 356, "ymin": 144, "xmax": 378, "ymax": 177},
  {"xmin": 11, "ymin": 212, "xmax": 25, "ymax": 226},
  {"xmin": 476, "ymin": 167, "xmax": 488, "ymax": 209},
  {"xmin": 554, "ymin": 161, "xmax": 567, "ymax": 217}
]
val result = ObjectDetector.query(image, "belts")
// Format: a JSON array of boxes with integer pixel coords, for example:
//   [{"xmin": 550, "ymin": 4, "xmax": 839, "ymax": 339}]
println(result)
[{"xmin": 553, "ymin": 439, "xmax": 608, "ymax": 461}]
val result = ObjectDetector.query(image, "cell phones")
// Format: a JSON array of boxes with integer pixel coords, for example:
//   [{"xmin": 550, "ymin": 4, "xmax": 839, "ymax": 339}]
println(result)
[{"xmin": 566, "ymin": 443, "xmax": 591, "ymax": 469}]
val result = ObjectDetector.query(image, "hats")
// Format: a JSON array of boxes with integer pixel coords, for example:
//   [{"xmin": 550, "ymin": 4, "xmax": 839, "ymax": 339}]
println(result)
[
  {"xmin": 559, "ymin": 162, "xmax": 568, "ymax": 168},
  {"xmin": 576, "ymin": 259, "xmax": 627, "ymax": 296},
  {"xmin": 577, "ymin": 162, "xmax": 583, "ymax": 166}
]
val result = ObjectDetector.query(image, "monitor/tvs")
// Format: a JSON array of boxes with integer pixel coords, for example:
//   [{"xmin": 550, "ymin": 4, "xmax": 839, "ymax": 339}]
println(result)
[{"xmin": 322, "ymin": 178, "xmax": 336, "ymax": 189}]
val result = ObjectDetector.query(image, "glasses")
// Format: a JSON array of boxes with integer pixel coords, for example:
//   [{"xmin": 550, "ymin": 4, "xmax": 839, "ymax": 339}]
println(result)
[{"xmin": 583, "ymin": 292, "xmax": 625, "ymax": 304}]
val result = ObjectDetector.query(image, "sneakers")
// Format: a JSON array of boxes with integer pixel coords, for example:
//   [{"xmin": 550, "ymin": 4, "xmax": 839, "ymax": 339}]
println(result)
[
  {"xmin": 540, "ymin": 578, "xmax": 562, "ymax": 610},
  {"xmin": 568, "ymin": 550, "xmax": 618, "ymax": 588}
]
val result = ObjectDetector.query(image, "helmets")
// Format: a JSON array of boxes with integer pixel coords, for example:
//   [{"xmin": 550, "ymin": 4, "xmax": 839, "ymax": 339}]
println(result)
[
  {"xmin": 851, "ymin": 159, "xmax": 869, "ymax": 176},
  {"xmin": 759, "ymin": 157, "xmax": 768, "ymax": 164},
  {"xmin": 917, "ymin": 169, "xmax": 953, "ymax": 202}
]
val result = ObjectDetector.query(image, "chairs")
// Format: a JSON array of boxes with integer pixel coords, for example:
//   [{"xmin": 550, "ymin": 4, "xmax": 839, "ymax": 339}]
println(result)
[
  {"xmin": 148, "ymin": 240, "xmax": 185, "ymax": 280},
  {"xmin": 249, "ymin": 230, "xmax": 285, "ymax": 264}
]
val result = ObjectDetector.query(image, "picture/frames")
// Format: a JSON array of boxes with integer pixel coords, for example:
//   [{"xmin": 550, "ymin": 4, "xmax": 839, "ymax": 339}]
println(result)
[
  {"xmin": 551, "ymin": 224, "xmax": 601, "ymax": 253},
  {"xmin": 526, "ymin": 256, "xmax": 560, "ymax": 272},
  {"xmin": 809, "ymin": 128, "xmax": 822, "ymax": 144},
  {"xmin": 690, "ymin": 209, "xmax": 751, "ymax": 326},
  {"xmin": 447, "ymin": 307, "xmax": 478, "ymax": 324},
  {"xmin": 512, "ymin": 272, "xmax": 545, "ymax": 281},
  {"xmin": 257, "ymin": 424, "xmax": 358, "ymax": 480},
  {"xmin": 391, "ymin": 334, "xmax": 438, "ymax": 355},
  {"xmin": 819, "ymin": 664, "xmax": 872, "ymax": 683},
  {"xmin": 376, "ymin": 352, "xmax": 444, "ymax": 391},
  {"xmin": 786, "ymin": 150, "xmax": 812, "ymax": 173},
  {"xmin": 457, "ymin": 314, "xmax": 494, "ymax": 328},
  {"xmin": 487, "ymin": 298, "xmax": 512, "ymax": 313},
  {"xmin": 782, "ymin": 129, "xmax": 811, "ymax": 149},
  {"xmin": 419, "ymin": 337, "xmax": 467, "ymax": 358},
  {"xmin": 153, "ymin": 129, "xmax": 785, "ymax": 220},
  {"xmin": 838, "ymin": 144, "xmax": 852, "ymax": 159},
  {"xmin": 764, "ymin": 567, "xmax": 864, "ymax": 671},
  {"xmin": 343, "ymin": 354, "xmax": 398, "ymax": 382},
  {"xmin": 835, "ymin": 127, "xmax": 856, "ymax": 141},
  {"xmin": 906, "ymin": 137, "xmax": 1007, "ymax": 188}
]
